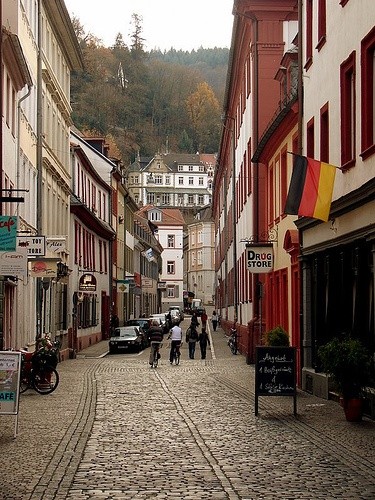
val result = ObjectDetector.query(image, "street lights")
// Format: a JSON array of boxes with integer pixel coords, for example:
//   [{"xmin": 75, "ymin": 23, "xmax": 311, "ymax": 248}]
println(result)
[{"xmin": 41, "ymin": 261, "xmax": 72, "ymax": 351}]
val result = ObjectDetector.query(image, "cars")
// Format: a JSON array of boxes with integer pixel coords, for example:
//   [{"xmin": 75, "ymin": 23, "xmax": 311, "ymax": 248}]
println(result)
[
  {"xmin": 168, "ymin": 310, "xmax": 180, "ymax": 326},
  {"xmin": 129, "ymin": 326, "xmax": 143, "ymax": 340},
  {"xmin": 149, "ymin": 317, "xmax": 163, "ymax": 338},
  {"xmin": 152, "ymin": 314, "xmax": 169, "ymax": 335},
  {"xmin": 108, "ymin": 327, "xmax": 143, "ymax": 354}
]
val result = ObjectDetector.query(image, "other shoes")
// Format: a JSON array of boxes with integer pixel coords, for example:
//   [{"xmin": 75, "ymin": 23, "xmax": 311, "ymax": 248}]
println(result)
[
  {"xmin": 170, "ymin": 361, "xmax": 172, "ymax": 364},
  {"xmin": 149, "ymin": 362, "xmax": 151, "ymax": 364},
  {"xmin": 158, "ymin": 354, "xmax": 160, "ymax": 359}
]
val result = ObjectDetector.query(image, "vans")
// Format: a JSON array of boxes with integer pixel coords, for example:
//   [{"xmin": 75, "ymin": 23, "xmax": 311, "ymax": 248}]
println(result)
[{"xmin": 168, "ymin": 305, "xmax": 185, "ymax": 321}]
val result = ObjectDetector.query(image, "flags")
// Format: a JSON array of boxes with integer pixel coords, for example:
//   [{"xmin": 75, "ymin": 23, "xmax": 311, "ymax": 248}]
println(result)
[{"xmin": 283, "ymin": 153, "xmax": 337, "ymax": 223}]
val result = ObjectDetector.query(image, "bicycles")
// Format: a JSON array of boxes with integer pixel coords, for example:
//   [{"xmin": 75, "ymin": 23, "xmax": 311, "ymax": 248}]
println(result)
[
  {"xmin": 20, "ymin": 357, "xmax": 61, "ymax": 394},
  {"xmin": 170, "ymin": 344, "xmax": 182, "ymax": 365},
  {"xmin": 149, "ymin": 341, "xmax": 161, "ymax": 368},
  {"xmin": 218, "ymin": 314, "xmax": 221, "ymax": 326}
]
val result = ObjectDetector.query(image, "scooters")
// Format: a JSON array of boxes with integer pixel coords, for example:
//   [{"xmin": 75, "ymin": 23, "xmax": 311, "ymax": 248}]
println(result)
[{"xmin": 224, "ymin": 327, "xmax": 241, "ymax": 355}]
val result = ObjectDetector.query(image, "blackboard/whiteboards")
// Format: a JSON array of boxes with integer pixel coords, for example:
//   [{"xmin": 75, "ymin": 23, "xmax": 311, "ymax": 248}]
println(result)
[{"xmin": 255, "ymin": 346, "xmax": 297, "ymax": 396}]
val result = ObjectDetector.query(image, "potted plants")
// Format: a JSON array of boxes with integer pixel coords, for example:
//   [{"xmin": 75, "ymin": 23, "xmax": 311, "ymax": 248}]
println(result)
[{"xmin": 317, "ymin": 333, "xmax": 371, "ymax": 422}]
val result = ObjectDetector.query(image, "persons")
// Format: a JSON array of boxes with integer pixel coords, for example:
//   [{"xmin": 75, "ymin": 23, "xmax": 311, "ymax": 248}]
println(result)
[
  {"xmin": 185, "ymin": 321, "xmax": 198, "ymax": 359},
  {"xmin": 191, "ymin": 310, "xmax": 218, "ymax": 331},
  {"xmin": 147, "ymin": 320, "xmax": 164, "ymax": 365},
  {"xmin": 198, "ymin": 327, "xmax": 211, "ymax": 360},
  {"xmin": 166, "ymin": 322, "xmax": 183, "ymax": 364}
]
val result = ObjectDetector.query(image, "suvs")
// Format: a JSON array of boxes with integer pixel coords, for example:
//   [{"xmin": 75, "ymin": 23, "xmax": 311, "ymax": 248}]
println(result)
[{"xmin": 127, "ymin": 320, "xmax": 149, "ymax": 348}]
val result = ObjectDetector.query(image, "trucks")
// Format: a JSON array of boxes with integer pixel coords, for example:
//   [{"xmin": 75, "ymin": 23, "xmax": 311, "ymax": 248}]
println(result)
[{"xmin": 186, "ymin": 298, "xmax": 206, "ymax": 316}]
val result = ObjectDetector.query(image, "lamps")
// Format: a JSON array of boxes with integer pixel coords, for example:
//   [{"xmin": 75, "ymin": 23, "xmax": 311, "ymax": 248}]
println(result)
[{"xmin": 56, "ymin": 262, "xmax": 68, "ymax": 282}]
